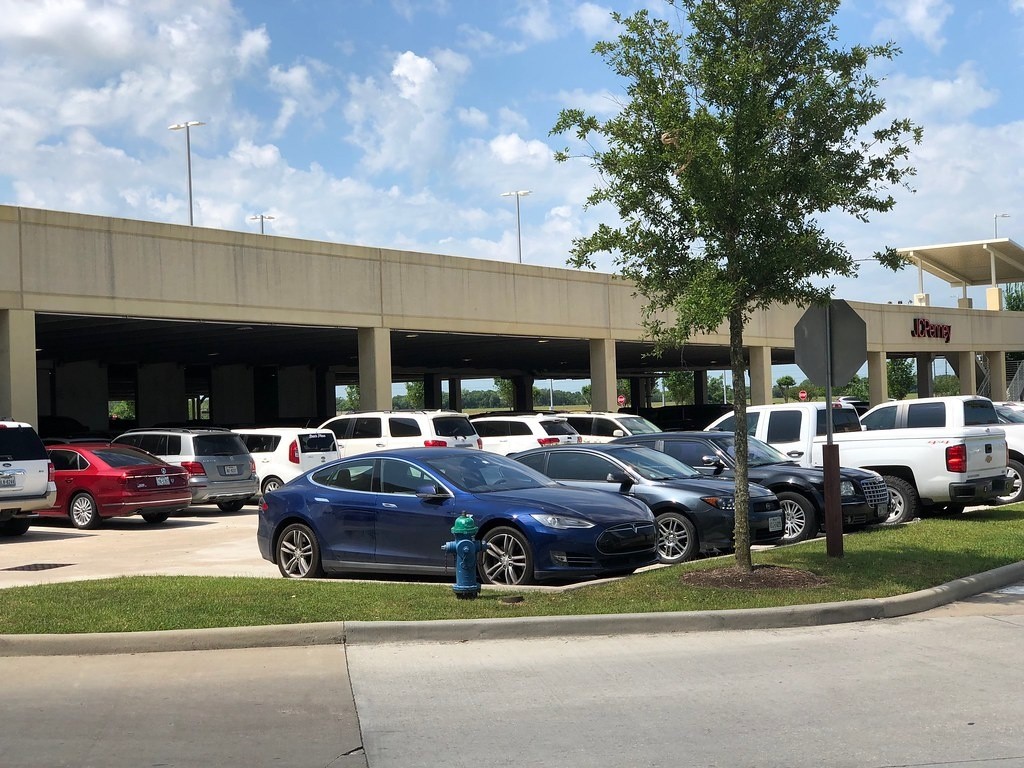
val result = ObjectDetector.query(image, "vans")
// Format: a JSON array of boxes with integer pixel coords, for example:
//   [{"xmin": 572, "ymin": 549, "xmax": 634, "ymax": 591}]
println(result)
[
  {"xmin": 555, "ymin": 410, "xmax": 656, "ymax": 444},
  {"xmin": 230, "ymin": 428, "xmax": 342, "ymax": 496}
]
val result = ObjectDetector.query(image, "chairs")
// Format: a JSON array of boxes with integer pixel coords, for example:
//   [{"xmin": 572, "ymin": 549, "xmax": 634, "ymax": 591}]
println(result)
[
  {"xmin": 386, "ymin": 463, "xmax": 419, "ymax": 496},
  {"xmin": 444, "ymin": 466, "xmax": 470, "ymax": 490},
  {"xmin": 336, "ymin": 469, "xmax": 354, "ymax": 490}
]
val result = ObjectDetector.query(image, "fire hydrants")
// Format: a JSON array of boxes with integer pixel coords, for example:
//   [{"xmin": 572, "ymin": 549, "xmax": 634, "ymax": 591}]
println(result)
[{"xmin": 441, "ymin": 512, "xmax": 492, "ymax": 600}]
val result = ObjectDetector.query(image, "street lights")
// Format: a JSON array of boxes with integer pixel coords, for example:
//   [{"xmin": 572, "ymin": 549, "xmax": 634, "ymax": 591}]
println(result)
[
  {"xmin": 250, "ymin": 214, "xmax": 275, "ymax": 235},
  {"xmin": 168, "ymin": 121, "xmax": 206, "ymax": 226},
  {"xmin": 994, "ymin": 213, "xmax": 1010, "ymax": 239},
  {"xmin": 500, "ymin": 190, "xmax": 532, "ymax": 263}
]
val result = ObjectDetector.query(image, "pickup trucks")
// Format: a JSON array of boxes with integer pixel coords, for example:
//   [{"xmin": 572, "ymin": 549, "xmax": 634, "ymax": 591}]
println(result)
[
  {"xmin": 858, "ymin": 396, "xmax": 1024, "ymax": 506},
  {"xmin": 703, "ymin": 400, "xmax": 1016, "ymax": 526}
]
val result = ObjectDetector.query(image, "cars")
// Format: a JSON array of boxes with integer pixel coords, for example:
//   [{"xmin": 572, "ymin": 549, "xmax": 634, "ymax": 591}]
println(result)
[
  {"xmin": 990, "ymin": 401, "xmax": 1024, "ymax": 424},
  {"xmin": 257, "ymin": 447, "xmax": 663, "ymax": 585},
  {"xmin": 605, "ymin": 432, "xmax": 893, "ymax": 545},
  {"xmin": 453, "ymin": 414, "xmax": 584, "ymax": 458},
  {"xmin": 479, "ymin": 442, "xmax": 785, "ymax": 564},
  {"xmin": 32, "ymin": 442, "xmax": 193, "ymax": 530}
]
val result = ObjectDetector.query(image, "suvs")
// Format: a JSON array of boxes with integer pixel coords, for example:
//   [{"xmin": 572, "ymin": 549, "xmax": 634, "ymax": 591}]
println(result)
[
  {"xmin": 0, "ymin": 417, "xmax": 58, "ymax": 536},
  {"xmin": 110, "ymin": 426, "xmax": 261, "ymax": 516},
  {"xmin": 318, "ymin": 409, "xmax": 483, "ymax": 477}
]
